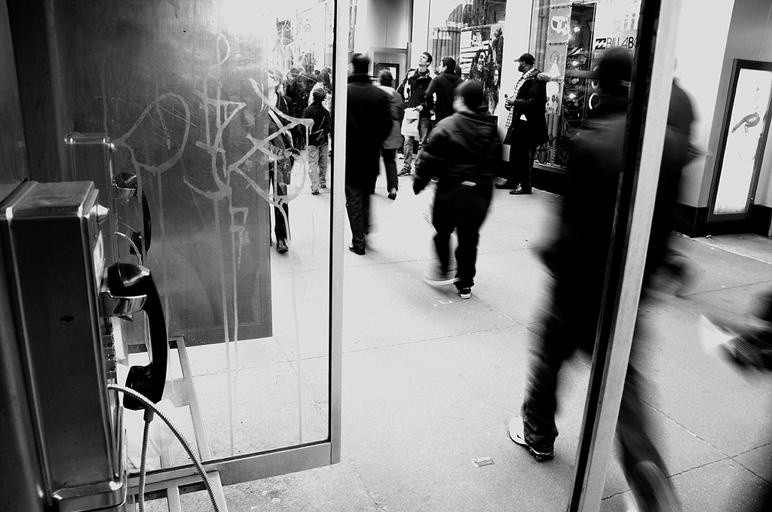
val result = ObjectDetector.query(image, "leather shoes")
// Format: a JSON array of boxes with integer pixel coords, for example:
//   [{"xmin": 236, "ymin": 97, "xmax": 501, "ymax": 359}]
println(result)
[
  {"xmin": 509, "ymin": 186, "xmax": 532, "ymax": 194},
  {"xmin": 349, "ymin": 245, "xmax": 365, "ymax": 255},
  {"xmin": 388, "ymin": 193, "xmax": 396, "ymax": 200},
  {"xmin": 276, "ymin": 239, "xmax": 288, "ymax": 252},
  {"xmin": 495, "ymin": 181, "xmax": 518, "ymax": 189}
]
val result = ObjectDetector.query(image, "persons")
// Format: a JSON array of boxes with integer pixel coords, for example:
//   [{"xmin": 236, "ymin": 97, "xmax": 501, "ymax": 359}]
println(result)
[
  {"xmin": 412, "ymin": 80, "xmax": 503, "ymax": 298},
  {"xmin": 269, "ymin": 68, "xmax": 300, "ymax": 253},
  {"xmin": 496, "ymin": 53, "xmax": 548, "ymax": 194},
  {"xmin": 650, "ymin": 80, "xmax": 698, "ymax": 296},
  {"xmin": 345, "ymin": 54, "xmax": 395, "ymax": 256},
  {"xmin": 277, "ymin": 67, "xmax": 333, "ymax": 195},
  {"xmin": 372, "ymin": 52, "xmax": 463, "ymax": 201},
  {"xmin": 507, "ymin": 48, "xmax": 700, "ymax": 512}
]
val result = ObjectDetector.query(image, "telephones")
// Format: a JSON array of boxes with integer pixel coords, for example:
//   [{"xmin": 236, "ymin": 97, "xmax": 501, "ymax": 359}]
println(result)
[{"xmin": 0, "ymin": 178, "xmax": 168, "ymax": 512}]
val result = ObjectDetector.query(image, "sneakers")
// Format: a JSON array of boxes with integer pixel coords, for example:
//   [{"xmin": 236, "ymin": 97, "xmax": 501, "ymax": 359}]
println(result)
[
  {"xmin": 508, "ymin": 415, "xmax": 554, "ymax": 462},
  {"xmin": 459, "ymin": 288, "xmax": 471, "ymax": 299},
  {"xmin": 397, "ymin": 168, "xmax": 411, "ymax": 176}
]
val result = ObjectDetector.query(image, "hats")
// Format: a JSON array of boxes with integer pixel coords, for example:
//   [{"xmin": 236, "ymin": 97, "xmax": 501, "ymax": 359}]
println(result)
[{"xmin": 514, "ymin": 54, "xmax": 535, "ymax": 65}]
